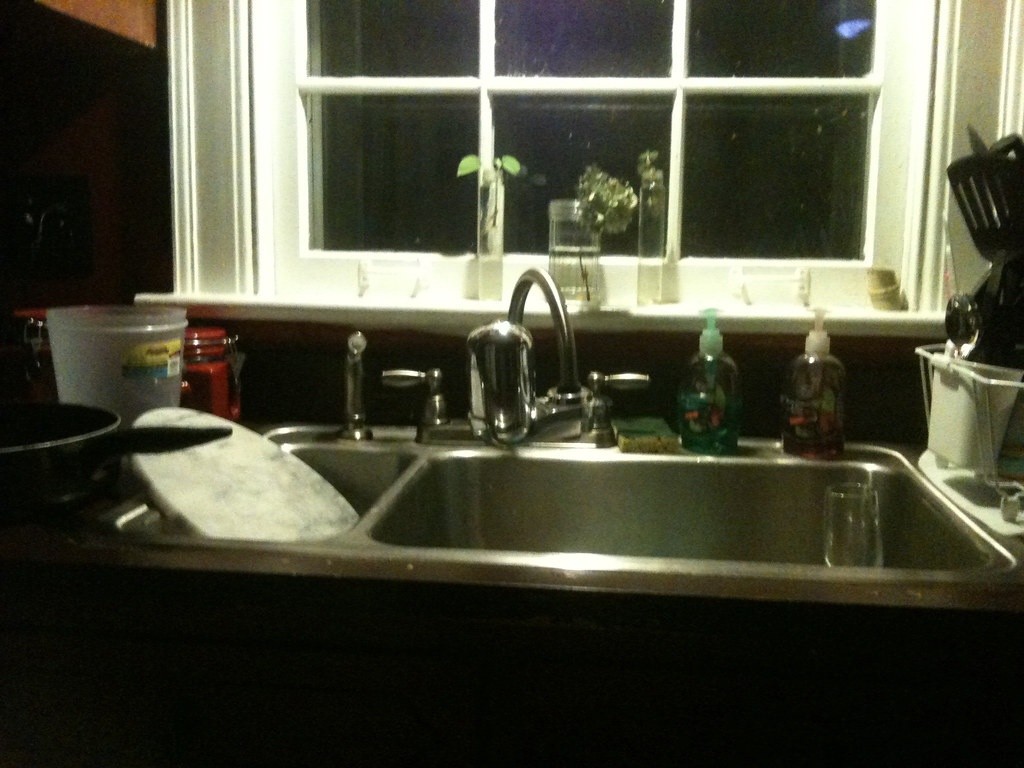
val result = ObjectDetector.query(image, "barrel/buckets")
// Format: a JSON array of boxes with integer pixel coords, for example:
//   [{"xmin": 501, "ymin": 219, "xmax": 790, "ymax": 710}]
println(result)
[{"xmin": 45, "ymin": 306, "xmax": 188, "ymax": 428}]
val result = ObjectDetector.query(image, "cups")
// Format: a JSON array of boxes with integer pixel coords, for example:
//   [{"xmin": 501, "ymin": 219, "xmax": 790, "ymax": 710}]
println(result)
[{"xmin": 820, "ymin": 480, "xmax": 885, "ymax": 568}]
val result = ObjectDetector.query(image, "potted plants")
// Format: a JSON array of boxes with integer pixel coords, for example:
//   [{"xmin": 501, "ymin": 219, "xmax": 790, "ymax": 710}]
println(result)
[
  {"xmin": 545, "ymin": 164, "xmax": 637, "ymax": 302},
  {"xmin": 637, "ymin": 142, "xmax": 668, "ymax": 302},
  {"xmin": 455, "ymin": 152, "xmax": 527, "ymax": 299}
]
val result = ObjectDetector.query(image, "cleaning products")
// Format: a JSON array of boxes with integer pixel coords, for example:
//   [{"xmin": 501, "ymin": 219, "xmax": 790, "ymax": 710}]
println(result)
[
  {"xmin": 678, "ymin": 307, "xmax": 740, "ymax": 455},
  {"xmin": 780, "ymin": 305, "xmax": 846, "ymax": 460}
]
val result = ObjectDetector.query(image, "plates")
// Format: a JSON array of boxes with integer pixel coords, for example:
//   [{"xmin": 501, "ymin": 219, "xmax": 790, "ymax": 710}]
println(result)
[{"xmin": 131, "ymin": 406, "xmax": 359, "ymax": 539}]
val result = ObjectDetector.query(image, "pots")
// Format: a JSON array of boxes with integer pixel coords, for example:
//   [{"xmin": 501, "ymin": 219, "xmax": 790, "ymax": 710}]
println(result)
[{"xmin": 0, "ymin": 396, "xmax": 232, "ymax": 516}]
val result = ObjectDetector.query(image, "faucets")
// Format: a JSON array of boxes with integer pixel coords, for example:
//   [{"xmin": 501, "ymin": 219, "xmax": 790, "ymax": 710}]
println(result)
[{"xmin": 462, "ymin": 264, "xmax": 586, "ymax": 448}]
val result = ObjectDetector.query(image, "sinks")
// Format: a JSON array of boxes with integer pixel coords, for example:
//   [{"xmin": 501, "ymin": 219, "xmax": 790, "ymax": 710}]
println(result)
[
  {"xmin": 341, "ymin": 431, "xmax": 1020, "ymax": 615},
  {"xmin": 84, "ymin": 419, "xmax": 427, "ymax": 579}
]
